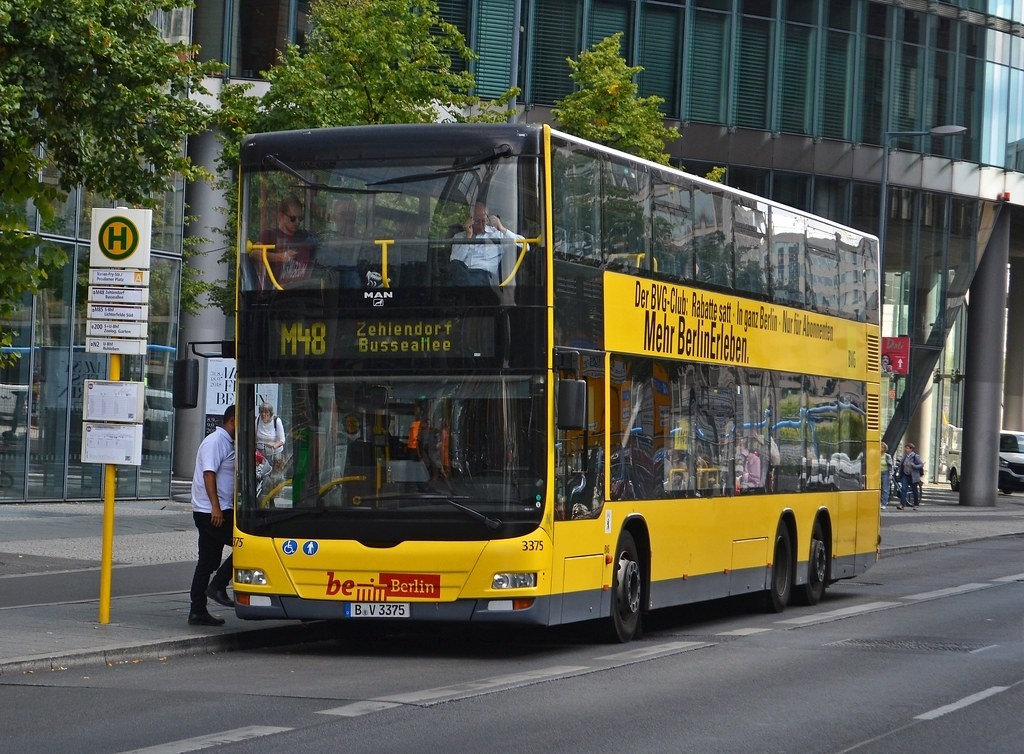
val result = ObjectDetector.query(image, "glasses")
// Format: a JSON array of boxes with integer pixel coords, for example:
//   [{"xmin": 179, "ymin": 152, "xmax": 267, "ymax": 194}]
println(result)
[
  {"xmin": 282, "ymin": 211, "xmax": 304, "ymax": 222},
  {"xmin": 336, "ymin": 211, "xmax": 356, "ymax": 216},
  {"xmin": 473, "ymin": 218, "xmax": 490, "ymax": 225}
]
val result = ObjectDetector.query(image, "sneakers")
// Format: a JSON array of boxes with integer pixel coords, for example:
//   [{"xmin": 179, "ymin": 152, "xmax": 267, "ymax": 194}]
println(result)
[
  {"xmin": 881, "ymin": 506, "xmax": 886, "ymax": 509},
  {"xmin": 913, "ymin": 505, "xmax": 920, "ymax": 510},
  {"xmin": 897, "ymin": 504, "xmax": 904, "ymax": 510},
  {"xmin": 364, "ymin": 271, "xmax": 391, "ymax": 290}
]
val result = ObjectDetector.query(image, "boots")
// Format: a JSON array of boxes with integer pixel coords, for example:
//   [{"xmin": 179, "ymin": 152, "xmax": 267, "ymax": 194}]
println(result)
[
  {"xmin": 204, "ymin": 578, "xmax": 235, "ymax": 607},
  {"xmin": 188, "ymin": 603, "xmax": 225, "ymax": 626}
]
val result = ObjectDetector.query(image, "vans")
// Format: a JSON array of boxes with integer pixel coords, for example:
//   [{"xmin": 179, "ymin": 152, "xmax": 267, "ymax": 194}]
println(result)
[{"xmin": 945, "ymin": 426, "xmax": 1024, "ymax": 495}]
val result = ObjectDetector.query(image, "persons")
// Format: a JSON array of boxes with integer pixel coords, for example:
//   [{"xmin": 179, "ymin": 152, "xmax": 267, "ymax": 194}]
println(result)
[
  {"xmin": 260, "ymin": 197, "xmax": 426, "ymax": 291},
  {"xmin": 440, "ymin": 201, "xmax": 530, "ymax": 305},
  {"xmin": 187, "ymin": 403, "xmax": 285, "ymax": 626},
  {"xmin": 343, "ymin": 415, "xmax": 361, "ymax": 441},
  {"xmin": 897, "ymin": 443, "xmax": 923, "ymax": 511},
  {"xmin": 735, "ymin": 436, "xmax": 760, "ymax": 488},
  {"xmin": 499, "ymin": 441, "xmax": 542, "ymax": 489},
  {"xmin": 881, "ymin": 442, "xmax": 892, "ymax": 510}
]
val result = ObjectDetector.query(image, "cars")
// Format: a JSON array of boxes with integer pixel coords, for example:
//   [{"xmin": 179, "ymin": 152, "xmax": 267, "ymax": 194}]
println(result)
[{"xmin": 431, "ymin": 444, "xmax": 489, "ymax": 478}]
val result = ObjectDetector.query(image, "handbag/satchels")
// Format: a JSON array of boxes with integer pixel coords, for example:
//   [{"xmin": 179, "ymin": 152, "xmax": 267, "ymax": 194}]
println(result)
[{"xmin": 913, "ymin": 453, "xmax": 924, "ymax": 477}]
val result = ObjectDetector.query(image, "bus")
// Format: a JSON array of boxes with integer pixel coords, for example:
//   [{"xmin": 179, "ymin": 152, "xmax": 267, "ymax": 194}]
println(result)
[
  {"xmin": 171, "ymin": 119, "xmax": 882, "ymax": 645},
  {"xmin": 0, "ymin": 344, "xmax": 181, "ymax": 441}
]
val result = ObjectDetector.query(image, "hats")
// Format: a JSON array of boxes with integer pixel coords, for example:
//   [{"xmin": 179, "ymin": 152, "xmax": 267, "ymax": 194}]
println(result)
[{"xmin": 255, "ymin": 450, "xmax": 264, "ymax": 464}]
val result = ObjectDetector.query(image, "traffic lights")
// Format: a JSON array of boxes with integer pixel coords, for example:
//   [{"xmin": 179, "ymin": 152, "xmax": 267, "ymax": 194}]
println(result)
[{"xmin": 415, "ymin": 394, "xmax": 431, "ymax": 424}]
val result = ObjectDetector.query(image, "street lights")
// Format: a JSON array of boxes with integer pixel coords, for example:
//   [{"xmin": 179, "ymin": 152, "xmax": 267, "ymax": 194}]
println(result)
[
  {"xmin": 370, "ymin": 385, "xmax": 388, "ymax": 446},
  {"xmin": 878, "ymin": 124, "xmax": 970, "ymax": 276}
]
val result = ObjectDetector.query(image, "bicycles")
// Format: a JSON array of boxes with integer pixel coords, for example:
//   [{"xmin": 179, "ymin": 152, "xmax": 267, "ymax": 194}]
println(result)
[{"xmin": 888, "ymin": 468, "xmax": 923, "ymax": 508}]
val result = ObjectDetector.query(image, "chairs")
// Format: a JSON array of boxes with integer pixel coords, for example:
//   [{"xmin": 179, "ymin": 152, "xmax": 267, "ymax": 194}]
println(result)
[{"xmin": 310, "ymin": 223, "xmax": 753, "ymax": 292}]
